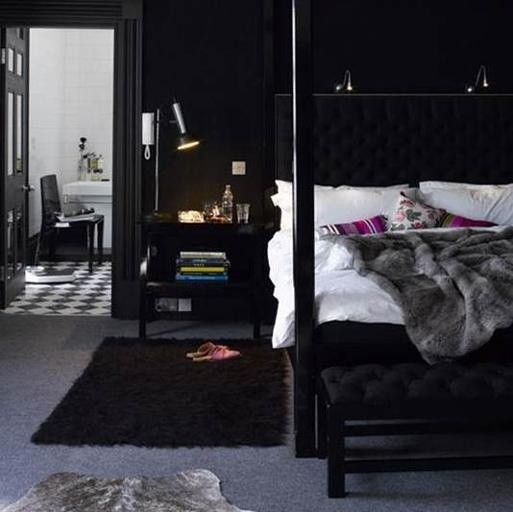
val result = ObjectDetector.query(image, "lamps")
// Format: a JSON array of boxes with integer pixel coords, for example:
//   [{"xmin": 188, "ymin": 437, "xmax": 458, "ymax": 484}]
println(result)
[
  {"xmin": 336, "ymin": 69, "xmax": 353, "ymax": 91},
  {"xmin": 467, "ymin": 65, "xmax": 489, "ymax": 93},
  {"xmin": 142, "ymin": 98, "xmax": 200, "ymax": 221}
]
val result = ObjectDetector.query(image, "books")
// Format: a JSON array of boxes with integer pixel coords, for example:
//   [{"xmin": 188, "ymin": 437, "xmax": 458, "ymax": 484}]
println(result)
[{"xmin": 174, "ymin": 250, "xmax": 231, "ymax": 281}]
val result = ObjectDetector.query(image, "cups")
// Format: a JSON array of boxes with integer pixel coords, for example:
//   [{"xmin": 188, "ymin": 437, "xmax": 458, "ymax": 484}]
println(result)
[{"xmin": 236, "ymin": 204, "xmax": 250, "ymax": 224}]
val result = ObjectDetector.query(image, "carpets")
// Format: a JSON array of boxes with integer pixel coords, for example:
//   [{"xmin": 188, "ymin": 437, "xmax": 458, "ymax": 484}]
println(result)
[
  {"xmin": 0, "ymin": 467, "xmax": 255, "ymax": 512},
  {"xmin": 25, "ymin": 275, "xmax": 75, "ymax": 284},
  {"xmin": 31, "ymin": 337, "xmax": 290, "ymax": 450}
]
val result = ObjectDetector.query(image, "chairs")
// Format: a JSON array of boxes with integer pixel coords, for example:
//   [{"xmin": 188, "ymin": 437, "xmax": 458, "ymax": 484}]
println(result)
[{"xmin": 33, "ymin": 174, "xmax": 104, "ymax": 274}]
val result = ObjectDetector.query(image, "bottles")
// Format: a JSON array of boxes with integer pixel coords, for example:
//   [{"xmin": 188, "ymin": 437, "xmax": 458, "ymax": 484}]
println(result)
[{"xmin": 222, "ymin": 184, "xmax": 233, "ymax": 220}]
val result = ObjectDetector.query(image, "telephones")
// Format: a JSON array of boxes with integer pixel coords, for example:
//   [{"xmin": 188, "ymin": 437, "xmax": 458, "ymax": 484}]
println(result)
[{"xmin": 141, "ymin": 112, "xmax": 155, "ymax": 145}]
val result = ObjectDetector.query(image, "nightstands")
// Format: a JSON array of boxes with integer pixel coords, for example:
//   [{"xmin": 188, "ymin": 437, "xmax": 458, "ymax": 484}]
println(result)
[{"xmin": 136, "ymin": 220, "xmax": 264, "ymax": 340}]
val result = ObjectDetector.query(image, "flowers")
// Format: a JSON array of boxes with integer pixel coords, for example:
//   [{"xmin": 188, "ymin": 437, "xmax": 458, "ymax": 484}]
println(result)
[{"xmin": 79, "ymin": 138, "xmax": 86, "ymax": 160}]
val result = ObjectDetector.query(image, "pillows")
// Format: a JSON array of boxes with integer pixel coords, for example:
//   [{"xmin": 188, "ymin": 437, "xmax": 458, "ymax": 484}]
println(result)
[{"xmin": 271, "ymin": 179, "xmax": 513, "ymax": 235}]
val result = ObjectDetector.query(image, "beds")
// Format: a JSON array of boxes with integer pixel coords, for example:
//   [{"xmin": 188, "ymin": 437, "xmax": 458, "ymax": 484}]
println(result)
[{"xmin": 262, "ymin": 0, "xmax": 513, "ymax": 458}]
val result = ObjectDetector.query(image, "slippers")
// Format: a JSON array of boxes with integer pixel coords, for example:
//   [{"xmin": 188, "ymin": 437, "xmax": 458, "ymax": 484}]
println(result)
[
  {"xmin": 185, "ymin": 341, "xmax": 228, "ymax": 359},
  {"xmin": 193, "ymin": 345, "xmax": 240, "ymax": 362}
]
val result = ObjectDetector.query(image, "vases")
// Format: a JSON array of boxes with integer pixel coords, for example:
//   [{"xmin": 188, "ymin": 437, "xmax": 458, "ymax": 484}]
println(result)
[{"xmin": 78, "ymin": 163, "xmax": 86, "ymax": 181}]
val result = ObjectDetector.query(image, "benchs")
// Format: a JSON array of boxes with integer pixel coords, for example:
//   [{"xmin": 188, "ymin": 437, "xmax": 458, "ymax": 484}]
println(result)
[{"xmin": 315, "ymin": 363, "xmax": 513, "ymax": 498}]
